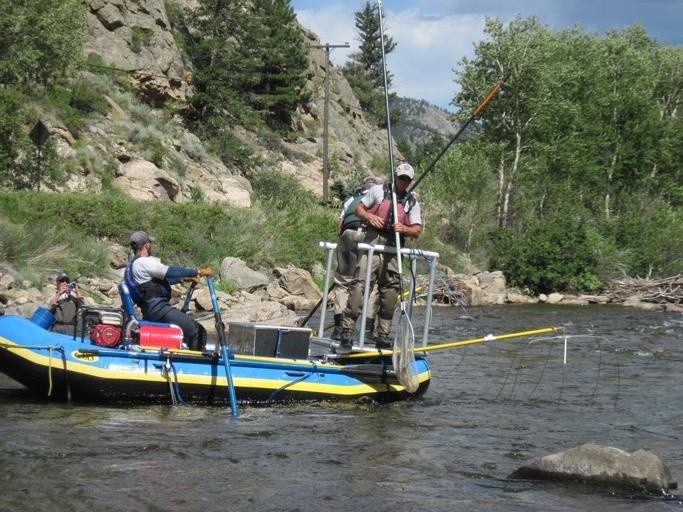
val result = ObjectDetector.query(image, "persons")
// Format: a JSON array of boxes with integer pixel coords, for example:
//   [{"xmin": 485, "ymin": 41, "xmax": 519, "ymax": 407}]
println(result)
[
  {"xmin": 333, "ymin": 163, "xmax": 422, "ymax": 354},
  {"xmin": 43, "ymin": 272, "xmax": 93, "ymax": 340},
  {"xmin": 330, "ymin": 175, "xmax": 382, "ymax": 344},
  {"xmin": 124, "ymin": 231, "xmax": 213, "ymax": 352}
]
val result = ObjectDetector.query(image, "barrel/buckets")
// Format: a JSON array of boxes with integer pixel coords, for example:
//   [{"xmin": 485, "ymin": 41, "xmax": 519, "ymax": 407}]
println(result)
[
  {"xmin": 31, "ymin": 307, "xmax": 56, "ymax": 330},
  {"xmin": 139, "ymin": 324, "xmax": 182, "ymax": 348}
]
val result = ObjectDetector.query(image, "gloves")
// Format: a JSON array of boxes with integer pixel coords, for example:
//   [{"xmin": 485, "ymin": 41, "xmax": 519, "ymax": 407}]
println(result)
[
  {"xmin": 184, "ymin": 276, "xmax": 199, "ymax": 284},
  {"xmin": 200, "ymin": 266, "xmax": 214, "ymax": 277}
]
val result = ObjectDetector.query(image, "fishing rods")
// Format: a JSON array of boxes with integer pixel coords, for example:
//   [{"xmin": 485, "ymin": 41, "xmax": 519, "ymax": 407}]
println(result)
[{"xmin": 300, "ymin": 60, "xmax": 520, "ymax": 326}]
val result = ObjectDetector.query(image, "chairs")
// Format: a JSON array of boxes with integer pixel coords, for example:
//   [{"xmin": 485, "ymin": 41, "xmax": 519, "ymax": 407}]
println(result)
[{"xmin": 117, "ymin": 283, "xmax": 182, "ymax": 347}]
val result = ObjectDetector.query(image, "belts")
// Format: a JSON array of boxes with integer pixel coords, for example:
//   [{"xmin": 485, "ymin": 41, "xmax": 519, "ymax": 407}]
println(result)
[{"xmin": 350, "ymin": 227, "xmax": 369, "ymax": 233}]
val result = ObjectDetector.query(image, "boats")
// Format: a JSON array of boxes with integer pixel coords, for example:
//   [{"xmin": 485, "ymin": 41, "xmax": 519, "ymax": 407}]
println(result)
[{"xmin": 1, "ymin": 241, "xmax": 439, "ymax": 401}]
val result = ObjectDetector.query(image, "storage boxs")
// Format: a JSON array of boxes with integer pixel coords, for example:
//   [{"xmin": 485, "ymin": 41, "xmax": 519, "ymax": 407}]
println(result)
[{"xmin": 226, "ymin": 320, "xmax": 313, "ymax": 359}]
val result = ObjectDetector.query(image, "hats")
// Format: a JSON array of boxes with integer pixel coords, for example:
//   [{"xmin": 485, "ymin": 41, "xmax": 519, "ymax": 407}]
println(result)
[
  {"xmin": 395, "ymin": 164, "xmax": 414, "ymax": 179},
  {"xmin": 362, "ymin": 176, "xmax": 384, "ymax": 184},
  {"xmin": 128, "ymin": 231, "xmax": 155, "ymax": 248},
  {"xmin": 56, "ymin": 272, "xmax": 69, "ymax": 282}
]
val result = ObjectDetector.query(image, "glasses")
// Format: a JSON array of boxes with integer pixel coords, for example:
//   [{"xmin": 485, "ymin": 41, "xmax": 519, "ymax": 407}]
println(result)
[{"xmin": 400, "ymin": 175, "xmax": 410, "ymax": 181}]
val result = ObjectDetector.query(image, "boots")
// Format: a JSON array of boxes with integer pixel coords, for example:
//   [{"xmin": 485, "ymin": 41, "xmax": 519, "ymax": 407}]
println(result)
[
  {"xmin": 375, "ymin": 332, "xmax": 393, "ymax": 349},
  {"xmin": 358, "ymin": 317, "xmax": 378, "ymax": 343},
  {"xmin": 340, "ymin": 328, "xmax": 354, "ymax": 350},
  {"xmin": 331, "ymin": 313, "xmax": 344, "ymax": 340}
]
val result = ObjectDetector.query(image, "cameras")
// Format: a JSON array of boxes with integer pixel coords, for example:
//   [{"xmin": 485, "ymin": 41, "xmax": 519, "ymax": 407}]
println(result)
[{"xmin": 67, "ymin": 283, "xmax": 76, "ymax": 288}]
